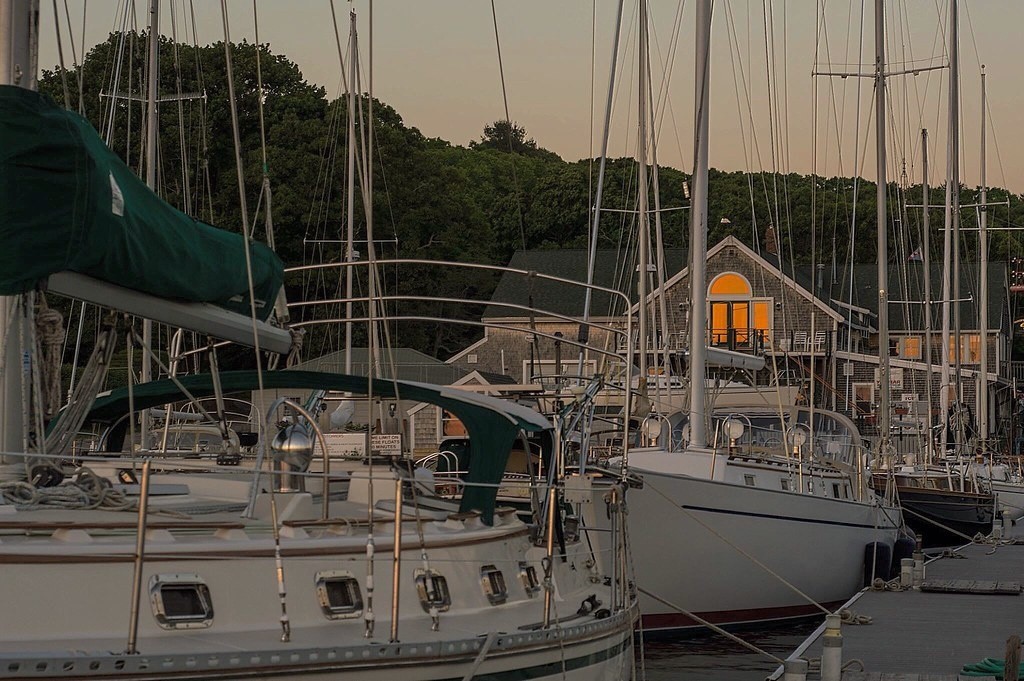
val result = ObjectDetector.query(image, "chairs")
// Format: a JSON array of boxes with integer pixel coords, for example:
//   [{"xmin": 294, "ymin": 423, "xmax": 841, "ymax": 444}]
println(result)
[{"xmin": 240, "ymin": 492, "xmax": 314, "ymax": 523}]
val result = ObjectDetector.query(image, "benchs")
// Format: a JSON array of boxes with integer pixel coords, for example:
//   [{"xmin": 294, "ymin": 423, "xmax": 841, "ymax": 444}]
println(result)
[{"xmin": 376, "ymin": 493, "xmax": 473, "ymax": 519}]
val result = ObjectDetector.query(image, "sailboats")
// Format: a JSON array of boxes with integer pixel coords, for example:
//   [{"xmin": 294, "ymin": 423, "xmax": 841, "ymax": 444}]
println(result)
[
  {"xmin": 868, "ymin": 1, "xmax": 1002, "ymax": 543},
  {"xmin": 564, "ymin": 0, "xmax": 903, "ymax": 632},
  {"xmin": 1, "ymin": 0, "xmax": 643, "ymax": 679}
]
